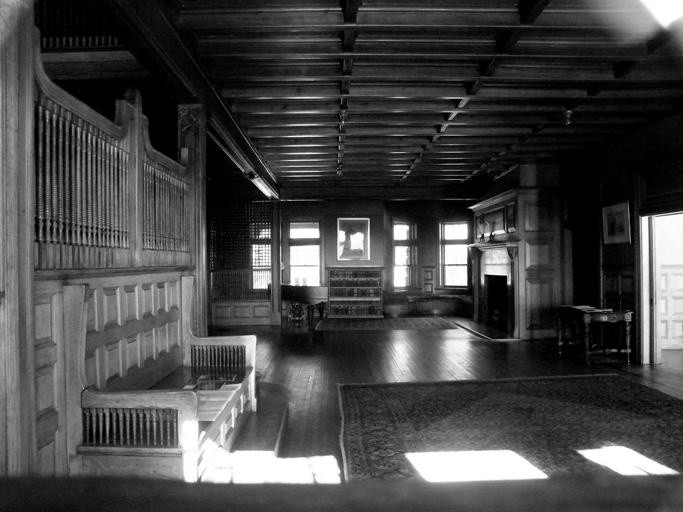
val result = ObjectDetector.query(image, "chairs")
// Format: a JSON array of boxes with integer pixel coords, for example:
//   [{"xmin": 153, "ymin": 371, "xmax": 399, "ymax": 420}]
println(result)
[{"xmin": 52, "ymin": 267, "xmax": 291, "ymax": 484}]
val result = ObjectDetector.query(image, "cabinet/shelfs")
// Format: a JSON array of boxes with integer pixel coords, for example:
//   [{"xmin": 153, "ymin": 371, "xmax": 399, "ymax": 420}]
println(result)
[{"xmin": 325, "ymin": 265, "xmax": 385, "ymax": 320}]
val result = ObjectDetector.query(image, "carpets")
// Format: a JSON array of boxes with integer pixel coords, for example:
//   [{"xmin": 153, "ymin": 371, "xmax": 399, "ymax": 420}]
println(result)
[
  {"xmin": 336, "ymin": 374, "xmax": 683, "ymax": 511},
  {"xmin": 315, "ymin": 316, "xmax": 458, "ymax": 330}
]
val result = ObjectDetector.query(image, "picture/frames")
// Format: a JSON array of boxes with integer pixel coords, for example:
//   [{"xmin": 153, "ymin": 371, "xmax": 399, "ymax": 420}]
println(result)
[
  {"xmin": 602, "ymin": 201, "xmax": 632, "ymax": 246},
  {"xmin": 337, "ymin": 217, "xmax": 371, "ymax": 262}
]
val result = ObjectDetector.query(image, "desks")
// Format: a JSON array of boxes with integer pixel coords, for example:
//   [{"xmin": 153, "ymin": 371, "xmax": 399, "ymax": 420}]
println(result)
[{"xmin": 551, "ymin": 303, "xmax": 633, "ymax": 368}]
